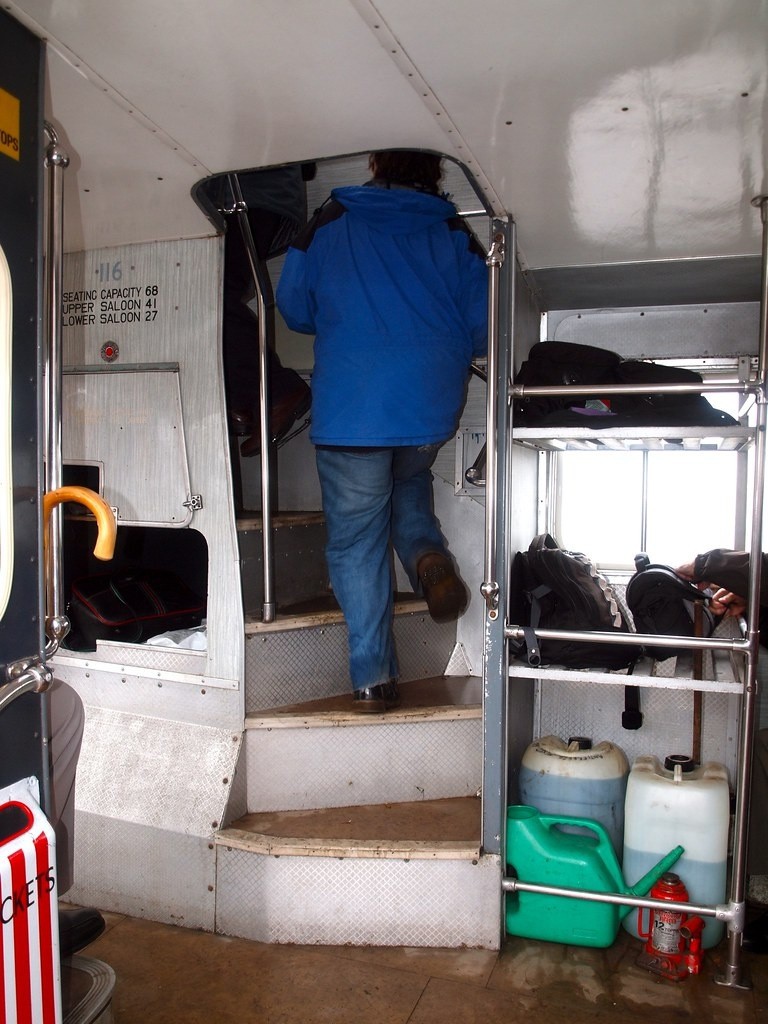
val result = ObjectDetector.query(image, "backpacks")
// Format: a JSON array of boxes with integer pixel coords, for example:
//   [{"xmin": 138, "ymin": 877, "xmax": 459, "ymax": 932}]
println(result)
[
  {"xmin": 626, "ymin": 554, "xmax": 728, "ymax": 662},
  {"xmin": 509, "ymin": 534, "xmax": 643, "ymax": 675},
  {"xmin": 614, "ymin": 361, "xmax": 703, "ymax": 409}
]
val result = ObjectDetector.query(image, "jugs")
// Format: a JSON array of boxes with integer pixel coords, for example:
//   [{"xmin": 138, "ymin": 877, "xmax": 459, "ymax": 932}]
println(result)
[{"xmin": 505, "ymin": 800, "xmax": 690, "ymax": 946}]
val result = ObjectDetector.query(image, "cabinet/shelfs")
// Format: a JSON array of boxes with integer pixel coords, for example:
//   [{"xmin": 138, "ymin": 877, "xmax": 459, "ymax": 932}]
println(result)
[{"xmin": 479, "ymin": 200, "xmax": 768, "ymax": 991}]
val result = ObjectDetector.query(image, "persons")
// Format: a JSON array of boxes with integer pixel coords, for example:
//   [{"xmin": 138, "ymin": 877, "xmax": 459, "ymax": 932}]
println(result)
[
  {"xmin": 203, "ymin": 159, "xmax": 320, "ymax": 458},
  {"xmin": 276, "ymin": 148, "xmax": 489, "ymax": 716}
]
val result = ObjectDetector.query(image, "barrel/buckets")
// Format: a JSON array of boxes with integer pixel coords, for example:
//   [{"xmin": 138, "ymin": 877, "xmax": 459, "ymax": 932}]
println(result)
[{"xmin": 507, "ymin": 735, "xmax": 732, "ymax": 947}]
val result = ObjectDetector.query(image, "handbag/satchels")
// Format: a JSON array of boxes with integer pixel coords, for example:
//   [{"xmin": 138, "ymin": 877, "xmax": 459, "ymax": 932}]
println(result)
[{"xmin": 62, "ymin": 564, "xmax": 207, "ymax": 652}]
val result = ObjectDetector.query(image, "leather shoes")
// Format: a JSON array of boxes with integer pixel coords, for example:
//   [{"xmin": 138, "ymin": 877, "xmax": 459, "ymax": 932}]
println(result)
[
  {"xmin": 228, "ymin": 368, "xmax": 312, "ymax": 457},
  {"xmin": 417, "ymin": 553, "xmax": 471, "ymax": 624},
  {"xmin": 354, "ymin": 679, "xmax": 400, "ymax": 714}
]
